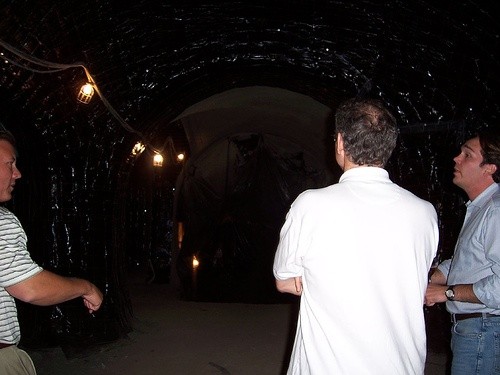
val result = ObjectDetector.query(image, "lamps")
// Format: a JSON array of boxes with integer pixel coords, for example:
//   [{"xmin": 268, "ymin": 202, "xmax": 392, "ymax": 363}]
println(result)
[
  {"xmin": 153, "ymin": 150, "xmax": 164, "ymax": 168},
  {"xmin": 132, "ymin": 140, "xmax": 148, "ymax": 155},
  {"xmin": 76, "ymin": 81, "xmax": 94, "ymax": 104},
  {"xmin": 176, "ymin": 150, "xmax": 185, "ymax": 161}
]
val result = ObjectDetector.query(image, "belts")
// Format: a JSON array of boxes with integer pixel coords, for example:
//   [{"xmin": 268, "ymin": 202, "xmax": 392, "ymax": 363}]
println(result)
[
  {"xmin": 452, "ymin": 313, "xmax": 500, "ymax": 321},
  {"xmin": 0, "ymin": 343, "xmax": 16, "ymax": 350}
]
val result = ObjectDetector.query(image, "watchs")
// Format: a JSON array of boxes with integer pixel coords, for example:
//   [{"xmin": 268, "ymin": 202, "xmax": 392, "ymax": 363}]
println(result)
[{"xmin": 445, "ymin": 285, "xmax": 455, "ymax": 301}]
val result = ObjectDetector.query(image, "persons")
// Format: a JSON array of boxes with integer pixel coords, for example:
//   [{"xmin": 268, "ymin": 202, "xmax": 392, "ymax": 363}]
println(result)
[
  {"xmin": 425, "ymin": 126, "xmax": 500, "ymax": 375},
  {"xmin": 272, "ymin": 102, "xmax": 440, "ymax": 375},
  {"xmin": 0, "ymin": 128, "xmax": 103, "ymax": 375}
]
